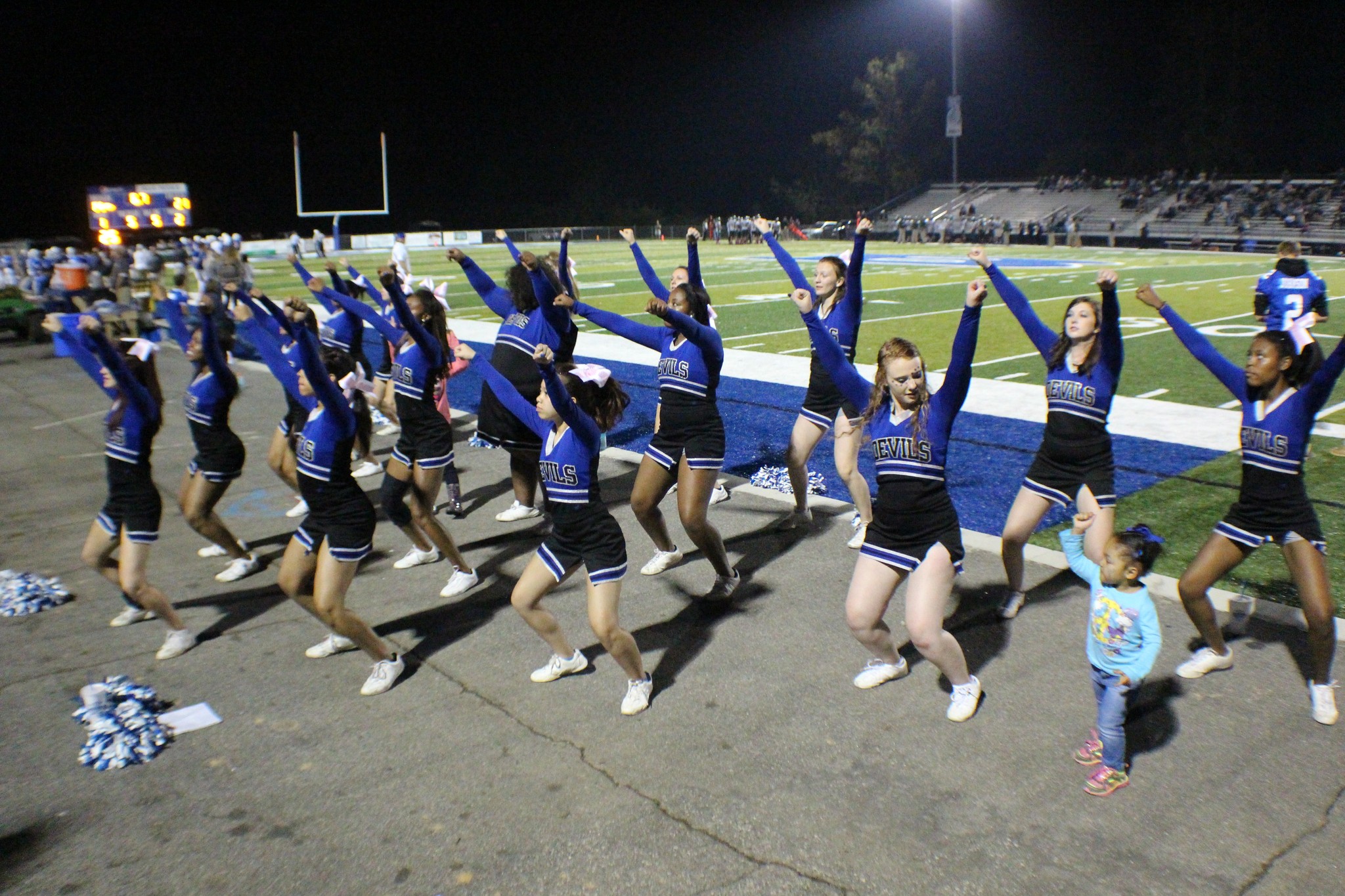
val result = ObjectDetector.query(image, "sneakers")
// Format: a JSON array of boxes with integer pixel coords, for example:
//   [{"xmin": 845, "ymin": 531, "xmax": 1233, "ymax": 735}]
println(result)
[
  {"xmin": 530, "ymin": 648, "xmax": 588, "ymax": 682},
  {"xmin": 393, "ymin": 544, "xmax": 439, "ymax": 569},
  {"xmin": 1339, "ymin": 699, "xmax": 1340, "ymax": 700},
  {"xmin": 777, "ymin": 506, "xmax": 812, "ymax": 531},
  {"xmin": 349, "ymin": 457, "xmax": 383, "ymax": 478},
  {"xmin": 946, "ymin": 674, "xmax": 981, "ymax": 722},
  {"xmin": 305, "ymin": 632, "xmax": 360, "ymax": 658},
  {"xmin": 708, "ymin": 484, "xmax": 728, "ymax": 505},
  {"xmin": 1308, "ymin": 678, "xmax": 1341, "ymax": 724},
  {"xmin": 1073, "ymin": 730, "xmax": 1103, "ymax": 766},
  {"xmin": 110, "ymin": 607, "xmax": 156, "ymax": 627},
  {"xmin": 1176, "ymin": 646, "xmax": 1233, "ymax": 678},
  {"xmin": 1083, "ymin": 764, "xmax": 1130, "ymax": 796},
  {"xmin": 285, "ymin": 496, "xmax": 309, "ymax": 517},
  {"xmin": 156, "ymin": 630, "xmax": 196, "ymax": 659},
  {"xmin": 440, "ymin": 565, "xmax": 478, "ymax": 598},
  {"xmin": 665, "ymin": 483, "xmax": 678, "ymax": 495},
  {"xmin": 352, "ymin": 652, "xmax": 404, "ymax": 694},
  {"xmin": 214, "ymin": 553, "xmax": 259, "ymax": 582},
  {"xmin": 854, "ymin": 655, "xmax": 908, "ymax": 688},
  {"xmin": 495, "ymin": 500, "xmax": 540, "ymax": 521},
  {"xmin": 705, "ymin": 567, "xmax": 740, "ymax": 600},
  {"xmin": 847, "ymin": 522, "xmax": 867, "ymax": 548},
  {"xmin": 641, "ymin": 545, "xmax": 683, "ymax": 575},
  {"xmin": 997, "ymin": 592, "xmax": 1025, "ymax": 618},
  {"xmin": 198, "ymin": 539, "xmax": 248, "ymax": 558},
  {"xmin": 376, "ymin": 425, "xmax": 402, "ymax": 436},
  {"xmin": 620, "ymin": 671, "xmax": 653, "ymax": 716}
]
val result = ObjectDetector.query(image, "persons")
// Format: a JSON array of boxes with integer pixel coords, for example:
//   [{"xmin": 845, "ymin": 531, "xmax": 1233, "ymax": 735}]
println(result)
[
  {"xmin": 223, "ymin": 282, "xmax": 320, "ymax": 519},
  {"xmin": 286, "ymin": 252, "xmax": 384, "ymax": 480},
  {"xmin": 340, "ymin": 257, "xmax": 412, "ymax": 438},
  {"xmin": 553, "ymin": 282, "xmax": 742, "ymax": 604},
  {"xmin": 1, "ymin": 229, "xmax": 417, "ymax": 347},
  {"xmin": 445, "ymin": 245, "xmax": 578, "ymax": 522},
  {"xmin": 389, "ymin": 277, "xmax": 472, "ymax": 517},
  {"xmin": 230, "ymin": 295, "xmax": 407, "ymax": 696},
  {"xmin": 40, "ymin": 312, "xmax": 197, "ymax": 661},
  {"xmin": 784, "ymin": 279, "xmax": 991, "ymax": 723},
  {"xmin": 967, "ymin": 245, "xmax": 1127, "ymax": 618},
  {"xmin": 307, "ymin": 266, "xmax": 479, "ymax": 599},
  {"xmin": 452, "ymin": 341, "xmax": 655, "ymax": 718},
  {"xmin": 1252, "ymin": 240, "xmax": 1330, "ymax": 333},
  {"xmin": 149, "ymin": 281, "xmax": 262, "ymax": 583},
  {"xmin": 534, "ymin": 142, "xmax": 1345, "ymax": 264},
  {"xmin": 1136, "ymin": 283, "xmax": 1345, "ymax": 727},
  {"xmin": 753, "ymin": 214, "xmax": 874, "ymax": 548},
  {"xmin": 492, "ymin": 225, "xmax": 580, "ymax": 367},
  {"xmin": 618, "ymin": 226, "xmax": 732, "ymax": 506},
  {"xmin": 1058, "ymin": 510, "xmax": 1166, "ymax": 797}
]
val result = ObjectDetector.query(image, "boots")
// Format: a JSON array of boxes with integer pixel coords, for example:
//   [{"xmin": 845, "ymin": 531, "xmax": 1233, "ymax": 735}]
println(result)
[{"xmin": 446, "ymin": 484, "xmax": 463, "ymax": 516}]
